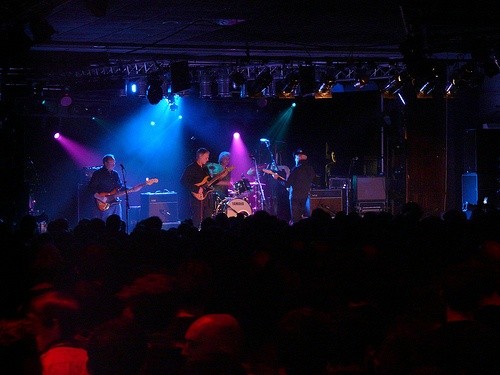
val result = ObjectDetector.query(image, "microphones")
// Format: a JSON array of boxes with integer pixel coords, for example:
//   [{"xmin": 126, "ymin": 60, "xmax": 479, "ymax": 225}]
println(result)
[
  {"xmin": 119, "ymin": 164, "xmax": 125, "ymax": 169},
  {"xmin": 260, "ymin": 138, "xmax": 269, "ymax": 142}
]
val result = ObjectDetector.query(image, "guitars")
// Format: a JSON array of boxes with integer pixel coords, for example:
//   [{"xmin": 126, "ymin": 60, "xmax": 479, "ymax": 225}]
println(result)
[
  {"xmin": 262, "ymin": 168, "xmax": 285, "ymax": 182},
  {"xmin": 191, "ymin": 165, "xmax": 236, "ymax": 202},
  {"xmin": 97, "ymin": 178, "xmax": 158, "ymax": 212}
]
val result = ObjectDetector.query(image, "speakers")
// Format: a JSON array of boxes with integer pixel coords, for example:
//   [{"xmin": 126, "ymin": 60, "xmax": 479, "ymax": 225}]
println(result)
[
  {"xmin": 461, "ymin": 173, "xmax": 500, "ymax": 219},
  {"xmin": 463, "ymin": 129, "xmax": 500, "ymax": 174}
]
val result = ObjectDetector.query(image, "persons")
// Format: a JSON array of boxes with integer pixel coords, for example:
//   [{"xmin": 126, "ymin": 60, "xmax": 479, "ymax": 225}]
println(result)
[
  {"xmin": 0, "ymin": 212, "xmax": 500, "ymax": 375},
  {"xmin": 272, "ymin": 148, "xmax": 315, "ymax": 218},
  {"xmin": 324, "ymin": 150, "xmax": 349, "ymax": 189},
  {"xmin": 87, "ymin": 154, "xmax": 142, "ymax": 223},
  {"xmin": 179, "ymin": 147, "xmax": 233, "ymax": 231}
]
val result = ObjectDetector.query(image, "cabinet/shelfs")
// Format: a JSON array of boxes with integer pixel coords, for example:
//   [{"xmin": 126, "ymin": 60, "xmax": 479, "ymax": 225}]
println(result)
[
  {"xmin": 141, "ymin": 192, "xmax": 179, "ymax": 224},
  {"xmin": 306, "ymin": 188, "xmax": 342, "ymax": 216}
]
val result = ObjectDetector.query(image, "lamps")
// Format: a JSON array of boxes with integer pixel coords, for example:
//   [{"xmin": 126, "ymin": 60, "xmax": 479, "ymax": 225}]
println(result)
[{"xmin": 24, "ymin": 56, "xmax": 500, "ymax": 111}]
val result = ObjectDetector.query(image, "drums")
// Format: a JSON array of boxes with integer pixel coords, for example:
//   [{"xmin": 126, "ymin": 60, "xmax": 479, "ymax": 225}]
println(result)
[
  {"xmin": 216, "ymin": 197, "xmax": 252, "ymax": 219},
  {"xmin": 233, "ymin": 177, "xmax": 252, "ymax": 196}
]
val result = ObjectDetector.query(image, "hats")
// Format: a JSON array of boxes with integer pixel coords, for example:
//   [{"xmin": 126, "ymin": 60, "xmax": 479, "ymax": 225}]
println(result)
[
  {"xmin": 116, "ymin": 272, "xmax": 172, "ymax": 302},
  {"xmin": 292, "ymin": 147, "xmax": 310, "ymax": 156}
]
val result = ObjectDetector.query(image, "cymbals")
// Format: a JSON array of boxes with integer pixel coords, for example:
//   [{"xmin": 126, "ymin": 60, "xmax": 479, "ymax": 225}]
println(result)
[{"xmin": 249, "ymin": 181, "xmax": 267, "ymax": 186}]
